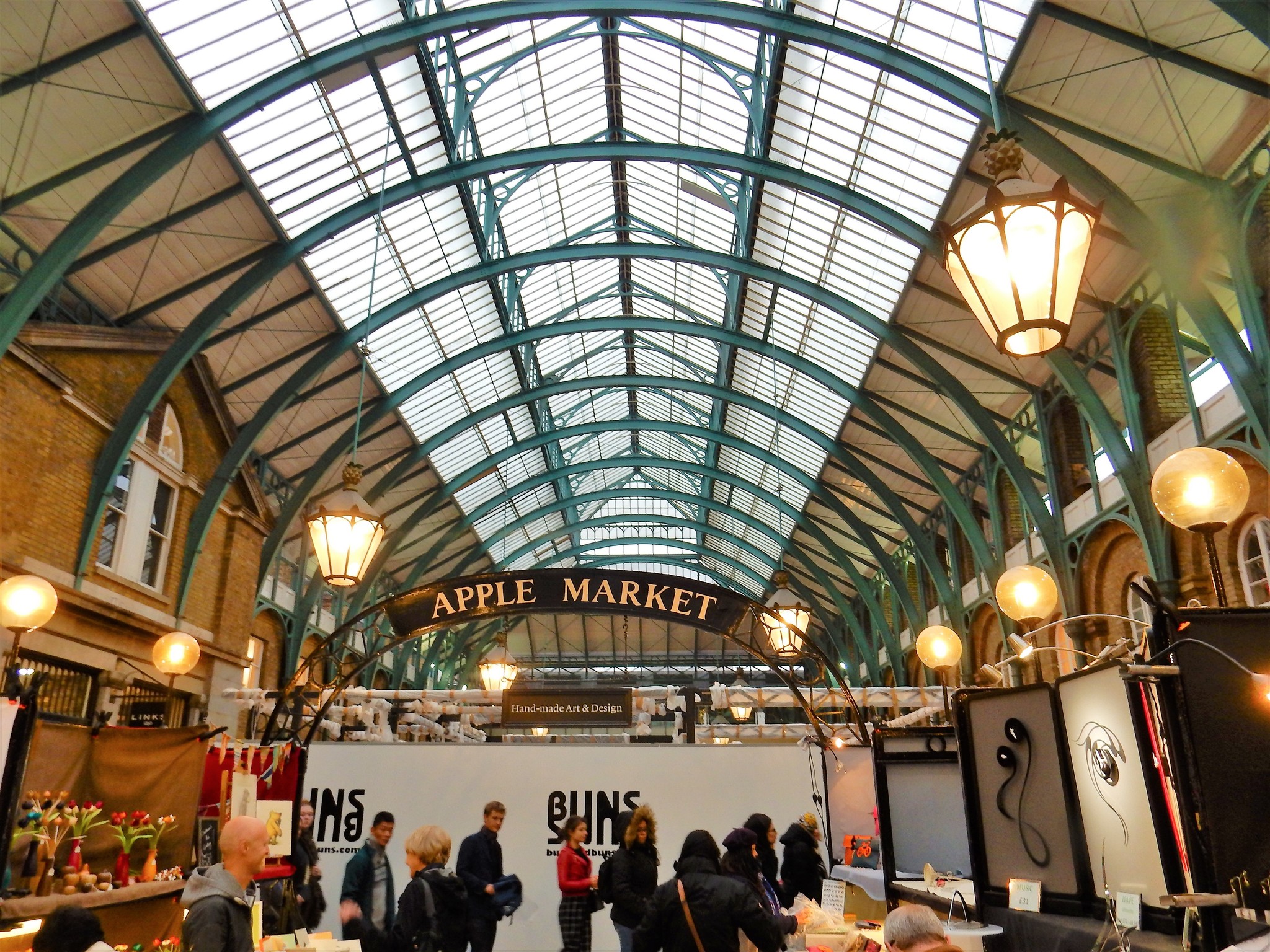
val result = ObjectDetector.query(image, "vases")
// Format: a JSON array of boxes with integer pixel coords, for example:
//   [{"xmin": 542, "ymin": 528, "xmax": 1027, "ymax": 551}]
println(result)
[{"xmin": 18, "ymin": 837, "xmax": 160, "ymax": 899}]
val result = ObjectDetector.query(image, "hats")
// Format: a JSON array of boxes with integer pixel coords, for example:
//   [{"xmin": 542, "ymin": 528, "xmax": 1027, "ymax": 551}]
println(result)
[
  {"xmin": 743, "ymin": 814, "xmax": 772, "ymax": 834},
  {"xmin": 32, "ymin": 902, "xmax": 104, "ymax": 952},
  {"xmin": 678, "ymin": 828, "xmax": 721, "ymax": 862},
  {"xmin": 798, "ymin": 812, "xmax": 817, "ymax": 830},
  {"xmin": 722, "ymin": 827, "xmax": 758, "ymax": 846}
]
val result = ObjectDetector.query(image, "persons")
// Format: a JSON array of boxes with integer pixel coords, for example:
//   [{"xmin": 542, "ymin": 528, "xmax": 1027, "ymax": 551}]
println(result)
[{"xmin": 23, "ymin": 783, "xmax": 981, "ymax": 952}]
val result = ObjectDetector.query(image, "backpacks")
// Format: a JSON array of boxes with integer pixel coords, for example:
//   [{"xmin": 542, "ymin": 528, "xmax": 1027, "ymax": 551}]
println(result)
[{"xmin": 597, "ymin": 847, "xmax": 615, "ymax": 904}]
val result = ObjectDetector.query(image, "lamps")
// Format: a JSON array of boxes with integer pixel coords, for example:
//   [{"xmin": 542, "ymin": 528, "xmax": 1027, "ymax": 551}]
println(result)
[
  {"xmin": 981, "ymin": 646, "xmax": 1107, "ymax": 685},
  {"xmin": 748, "ymin": 302, "xmax": 817, "ymax": 657},
  {"xmin": 797, "ymin": 730, "xmax": 846, "ymax": 773},
  {"xmin": 478, "ymin": 411, "xmax": 525, "ymax": 691},
  {"xmin": 1150, "ymin": 445, "xmax": 1257, "ymax": 610},
  {"xmin": 928, "ymin": 1, "xmax": 1106, "ymax": 365},
  {"xmin": 0, "ymin": 574, "xmax": 60, "ymax": 698},
  {"xmin": 150, "ymin": 631, "xmax": 201, "ymax": 728},
  {"xmin": 988, "ymin": 565, "xmax": 1061, "ymax": 684},
  {"xmin": 301, "ymin": 111, "xmax": 412, "ymax": 587},
  {"xmin": 1005, "ymin": 612, "xmax": 1154, "ymax": 662},
  {"xmin": 913, "ymin": 625, "xmax": 961, "ymax": 727}
]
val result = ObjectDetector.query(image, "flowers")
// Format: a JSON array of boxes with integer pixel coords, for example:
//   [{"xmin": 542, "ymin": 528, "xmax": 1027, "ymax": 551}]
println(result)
[
  {"xmin": 9, "ymin": 785, "xmax": 181, "ymax": 855},
  {"xmin": 152, "ymin": 862, "xmax": 183, "ymax": 884}
]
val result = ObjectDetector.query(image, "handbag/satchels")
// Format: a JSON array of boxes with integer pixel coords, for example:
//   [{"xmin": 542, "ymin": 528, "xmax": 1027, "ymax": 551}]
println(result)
[
  {"xmin": 402, "ymin": 875, "xmax": 442, "ymax": 952},
  {"xmin": 259, "ymin": 877, "xmax": 313, "ymax": 936},
  {"xmin": 590, "ymin": 887, "xmax": 605, "ymax": 912},
  {"xmin": 473, "ymin": 874, "xmax": 523, "ymax": 922}
]
